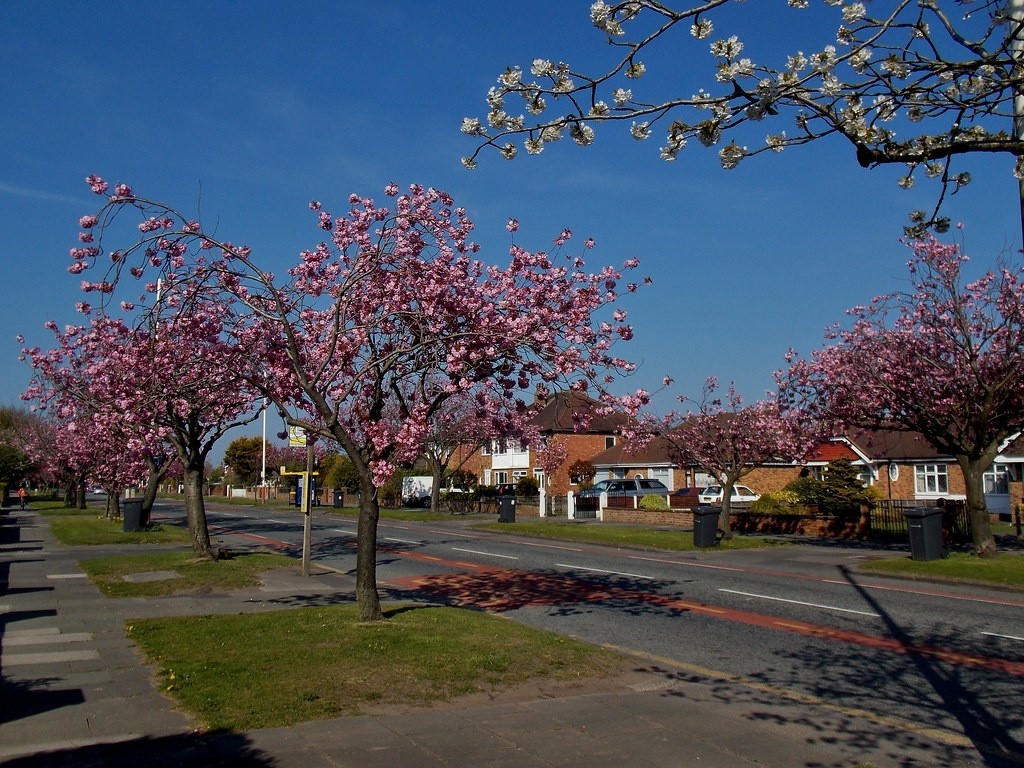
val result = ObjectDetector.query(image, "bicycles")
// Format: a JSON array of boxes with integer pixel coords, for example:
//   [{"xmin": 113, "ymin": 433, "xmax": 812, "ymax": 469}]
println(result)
[{"xmin": 21, "ymin": 496, "xmax": 27, "ymax": 509}]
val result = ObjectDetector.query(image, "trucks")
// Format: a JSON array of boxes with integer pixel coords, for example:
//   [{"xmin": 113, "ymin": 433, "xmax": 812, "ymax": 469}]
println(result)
[{"xmin": 402, "ymin": 476, "xmax": 475, "ymax": 508}]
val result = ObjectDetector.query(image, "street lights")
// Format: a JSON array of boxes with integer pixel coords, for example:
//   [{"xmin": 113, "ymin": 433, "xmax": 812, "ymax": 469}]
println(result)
[{"xmin": 156, "ymin": 278, "xmax": 181, "ymax": 338}]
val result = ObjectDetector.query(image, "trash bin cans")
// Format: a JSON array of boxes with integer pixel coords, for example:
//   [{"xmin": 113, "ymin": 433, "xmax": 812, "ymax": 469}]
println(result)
[
  {"xmin": 691, "ymin": 506, "xmax": 722, "ymax": 548},
  {"xmin": 122, "ymin": 497, "xmax": 145, "ymax": 533},
  {"xmin": 331, "ymin": 491, "xmax": 344, "ymax": 507},
  {"xmin": 903, "ymin": 506, "xmax": 951, "ymax": 562},
  {"xmin": 498, "ymin": 496, "xmax": 517, "ymax": 522}
]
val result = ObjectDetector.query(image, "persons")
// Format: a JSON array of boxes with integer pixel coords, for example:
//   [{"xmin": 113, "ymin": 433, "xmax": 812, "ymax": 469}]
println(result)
[{"xmin": 18, "ymin": 488, "xmax": 29, "ymax": 509}]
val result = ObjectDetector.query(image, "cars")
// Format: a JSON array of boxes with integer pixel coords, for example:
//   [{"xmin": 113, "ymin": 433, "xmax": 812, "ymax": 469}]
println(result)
[
  {"xmin": 668, "ymin": 487, "xmax": 718, "ymax": 498},
  {"xmin": 699, "ymin": 484, "xmax": 761, "ymax": 506},
  {"xmin": 94, "ymin": 486, "xmax": 107, "ymax": 495},
  {"xmin": 492, "ymin": 483, "xmax": 520, "ymax": 496}
]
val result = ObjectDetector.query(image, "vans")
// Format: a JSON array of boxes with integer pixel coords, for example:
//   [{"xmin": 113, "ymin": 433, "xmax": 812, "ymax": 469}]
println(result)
[{"xmin": 580, "ymin": 479, "xmax": 669, "ymax": 497}]
